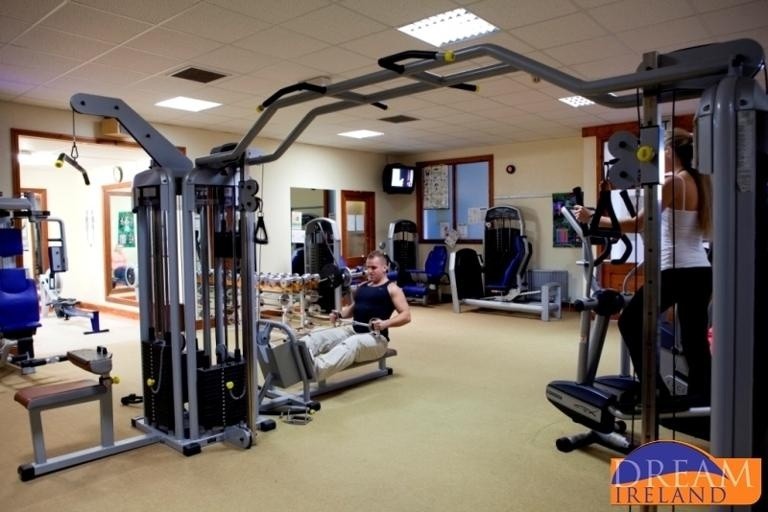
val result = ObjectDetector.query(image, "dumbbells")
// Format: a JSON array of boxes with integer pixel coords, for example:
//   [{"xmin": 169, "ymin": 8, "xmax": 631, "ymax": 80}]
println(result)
[{"xmin": 260, "ymin": 273, "xmax": 321, "ymax": 288}]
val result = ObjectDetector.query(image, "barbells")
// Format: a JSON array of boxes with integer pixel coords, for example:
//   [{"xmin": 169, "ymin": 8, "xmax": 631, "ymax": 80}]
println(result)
[{"xmin": 255, "ymin": 289, "xmax": 325, "ymax": 329}]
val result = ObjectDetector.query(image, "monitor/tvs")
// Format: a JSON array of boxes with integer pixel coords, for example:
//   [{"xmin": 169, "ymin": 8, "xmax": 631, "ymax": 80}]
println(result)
[
  {"xmin": 381, "ymin": 163, "xmax": 417, "ymax": 195},
  {"xmin": 561, "ymin": 205, "xmax": 610, "ymax": 245}
]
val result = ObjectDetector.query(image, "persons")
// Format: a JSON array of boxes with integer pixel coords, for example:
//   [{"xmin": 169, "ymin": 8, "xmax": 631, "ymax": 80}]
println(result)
[
  {"xmin": 571, "ymin": 126, "xmax": 712, "ymax": 422},
  {"xmin": 299, "ymin": 251, "xmax": 411, "ymax": 378}
]
